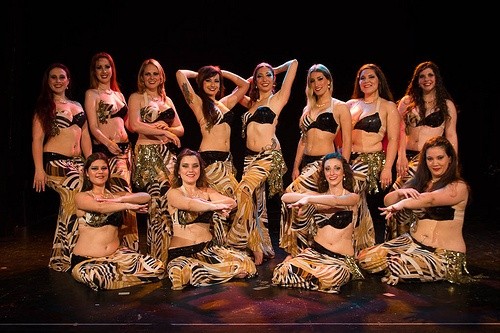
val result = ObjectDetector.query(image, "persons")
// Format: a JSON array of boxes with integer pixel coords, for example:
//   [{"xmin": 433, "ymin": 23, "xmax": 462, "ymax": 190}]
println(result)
[
  {"xmin": 271, "ymin": 153, "xmax": 363, "ymax": 294},
  {"xmin": 225, "ymin": 58, "xmax": 298, "ymax": 266},
  {"xmin": 127, "ymin": 58, "xmax": 185, "ymax": 261},
  {"xmin": 70, "ymin": 153, "xmax": 165, "ymax": 291},
  {"xmin": 385, "ymin": 61, "xmax": 458, "ymax": 242},
  {"xmin": 279, "ymin": 63, "xmax": 351, "ymax": 258},
  {"xmin": 358, "ymin": 135, "xmax": 469, "ymax": 283},
  {"xmin": 344, "ymin": 63, "xmax": 400, "ymax": 253},
  {"xmin": 85, "ymin": 53, "xmax": 140, "ymax": 253},
  {"xmin": 176, "ymin": 65, "xmax": 250, "ymax": 234},
  {"xmin": 167, "ymin": 149, "xmax": 258, "ymax": 290},
  {"xmin": 31, "ymin": 64, "xmax": 92, "ymax": 272}
]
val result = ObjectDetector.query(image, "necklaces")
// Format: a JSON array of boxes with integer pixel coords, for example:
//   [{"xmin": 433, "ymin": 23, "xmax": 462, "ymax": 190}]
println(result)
[
  {"xmin": 100, "ymin": 193, "xmax": 109, "ymax": 199},
  {"xmin": 315, "ymin": 103, "xmax": 325, "ymax": 108},
  {"xmin": 424, "ymin": 100, "xmax": 433, "ymax": 104},
  {"xmin": 363, "ymin": 98, "xmax": 377, "ymax": 104}
]
